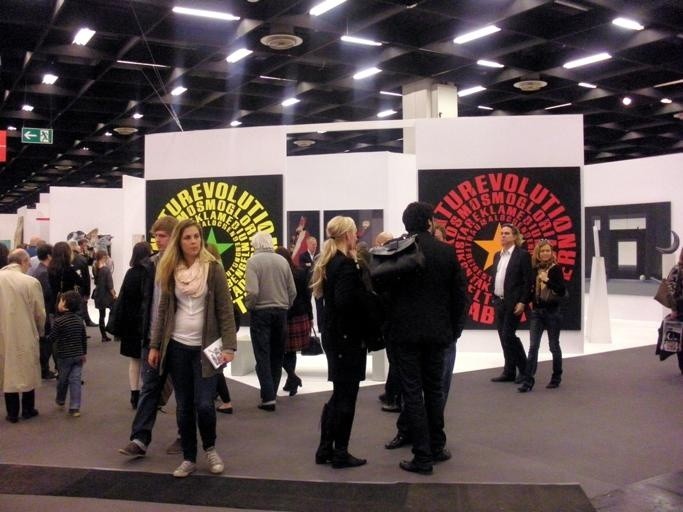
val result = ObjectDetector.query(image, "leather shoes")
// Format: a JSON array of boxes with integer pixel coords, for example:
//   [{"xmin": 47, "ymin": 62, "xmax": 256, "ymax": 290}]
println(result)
[
  {"xmin": 491, "ymin": 374, "xmax": 561, "ymax": 392},
  {"xmin": 316, "ymin": 394, "xmax": 451, "ymax": 474},
  {"xmin": 5, "ymin": 371, "xmax": 302, "ymax": 423}
]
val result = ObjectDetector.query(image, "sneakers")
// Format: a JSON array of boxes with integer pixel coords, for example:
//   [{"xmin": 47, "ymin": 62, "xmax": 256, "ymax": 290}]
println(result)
[
  {"xmin": 118, "ymin": 438, "xmax": 147, "ymax": 457},
  {"xmin": 204, "ymin": 447, "xmax": 224, "ymax": 474},
  {"xmin": 165, "ymin": 435, "xmax": 182, "ymax": 453},
  {"xmin": 172, "ymin": 460, "xmax": 197, "ymax": 478}
]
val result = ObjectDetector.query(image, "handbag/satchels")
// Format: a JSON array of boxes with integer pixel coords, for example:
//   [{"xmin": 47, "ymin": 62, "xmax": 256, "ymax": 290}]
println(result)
[
  {"xmin": 539, "ymin": 281, "xmax": 570, "ymax": 303},
  {"xmin": 368, "ymin": 233, "xmax": 427, "ymax": 294},
  {"xmin": 301, "ymin": 337, "xmax": 323, "ymax": 355},
  {"xmin": 104, "ymin": 299, "xmax": 120, "ymax": 337},
  {"xmin": 91, "ymin": 288, "xmax": 97, "ymax": 298}
]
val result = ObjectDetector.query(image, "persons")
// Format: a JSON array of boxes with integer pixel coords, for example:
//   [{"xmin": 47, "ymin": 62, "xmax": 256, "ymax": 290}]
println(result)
[
  {"xmin": 107, "ymin": 217, "xmax": 241, "ymax": 478},
  {"xmin": 488, "ymin": 224, "xmax": 532, "ymax": 382},
  {"xmin": 0, "ymin": 239, "xmax": 116, "ymax": 423},
  {"xmin": 517, "ymin": 239, "xmax": 569, "ymax": 392},
  {"xmin": 316, "ymin": 202, "xmax": 460, "ymax": 476},
  {"xmin": 244, "ymin": 231, "xmax": 318, "ymax": 411}
]
